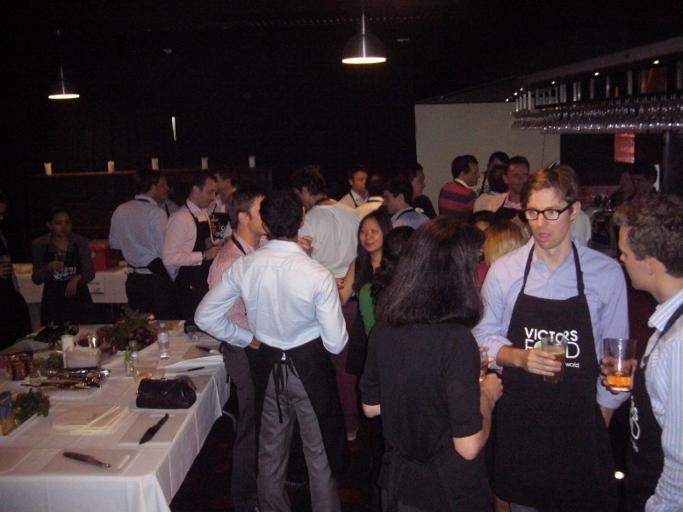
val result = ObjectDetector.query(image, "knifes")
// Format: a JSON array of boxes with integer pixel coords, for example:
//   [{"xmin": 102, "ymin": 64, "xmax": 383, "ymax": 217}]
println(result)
[
  {"xmin": 59, "ymin": 449, "xmax": 115, "ymax": 469},
  {"xmin": 138, "ymin": 412, "xmax": 171, "ymax": 445}
]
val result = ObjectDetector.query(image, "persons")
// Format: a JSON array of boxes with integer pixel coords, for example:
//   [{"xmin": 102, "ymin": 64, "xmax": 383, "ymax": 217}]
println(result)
[
  {"xmin": 107, "ymin": 170, "xmax": 170, "ymax": 319},
  {"xmin": 29, "ymin": 208, "xmax": 94, "ymax": 326},
  {"xmin": 207, "ymin": 193, "xmax": 268, "ymax": 509},
  {"xmin": 381, "ymin": 181, "xmax": 430, "ymax": 231},
  {"xmin": 474, "ymin": 166, "xmax": 629, "ymax": 512},
  {"xmin": 459, "ymin": 207, "xmax": 493, "ymax": 290},
  {"xmin": 438, "ymin": 155, "xmax": 481, "ymax": 214},
  {"xmin": 361, "ymin": 225, "xmax": 417, "ymax": 334},
  {"xmin": 290, "ymin": 172, "xmax": 362, "ymax": 443},
  {"xmin": 480, "ymin": 154, "xmax": 526, "ymax": 221},
  {"xmin": 2, "ymin": 202, "xmax": 31, "ymax": 338},
  {"xmin": 329, "ymin": 211, "xmax": 390, "ymax": 463},
  {"xmin": 354, "ymin": 173, "xmax": 382, "ymax": 218},
  {"xmin": 194, "ymin": 190, "xmax": 350, "ymax": 512},
  {"xmin": 331, "ymin": 170, "xmax": 367, "ymax": 206},
  {"xmin": 390, "ymin": 166, "xmax": 438, "ymax": 221},
  {"xmin": 475, "ymin": 151, "xmax": 506, "ymax": 195},
  {"xmin": 361, "ymin": 218, "xmax": 504, "ymax": 512},
  {"xmin": 163, "ymin": 172, "xmax": 220, "ymax": 320},
  {"xmin": 602, "ymin": 198, "xmax": 683, "ymax": 511},
  {"xmin": 471, "ymin": 168, "xmax": 506, "ymax": 215},
  {"xmin": 555, "ymin": 163, "xmax": 592, "ymax": 248},
  {"xmin": 609, "ymin": 173, "xmax": 633, "ymax": 213},
  {"xmin": 154, "ymin": 180, "xmax": 174, "ymax": 218},
  {"xmin": 210, "ymin": 169, "xmax": 236, "ymax": 229},
  {"xmin": 626, "ymin": 164, "xmax": 659, "ymax": 199},
  {"xmin": 480, "ymin": 222, "xmax": 525, "ymax": 267}
]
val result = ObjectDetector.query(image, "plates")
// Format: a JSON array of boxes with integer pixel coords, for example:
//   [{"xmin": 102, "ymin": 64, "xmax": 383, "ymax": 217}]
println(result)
[
  {"xmin": 117, "ymin": 339, "xmax": 162, "ymax": 359},
  {"xmin": 1, "ymin": 386, "xmax": 55, "ymax": 440}
]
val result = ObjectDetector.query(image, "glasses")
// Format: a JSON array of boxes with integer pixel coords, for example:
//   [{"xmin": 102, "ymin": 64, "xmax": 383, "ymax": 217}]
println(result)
[{"xmin": 523, "ymin": 203, "xmax": 572, "ymax": 222}]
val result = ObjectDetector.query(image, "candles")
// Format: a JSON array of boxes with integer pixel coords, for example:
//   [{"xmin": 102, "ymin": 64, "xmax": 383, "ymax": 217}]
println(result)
[
  {"xmin": 248, "ymin": 157, "xmax": 255, "ymax": 167},
  {"xmin": 108, "ymin": 161, "xmax": 114, "ymax": 172},
  {"xmin": 201, "ymin": 157, "xmax": 207, "ymax": 169},
  {"xmin": 152, "ymin": 158, "xmax": 158, "ymax": 170},
  {"xmin": 43, "ymin": 163, "xmax": 52, "ymax": 176}
]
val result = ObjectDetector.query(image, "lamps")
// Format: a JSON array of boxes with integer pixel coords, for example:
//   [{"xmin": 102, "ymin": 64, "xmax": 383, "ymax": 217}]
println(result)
[
  {"xmin": 47, "ymin": 23, "xmax": 80, "ymax": 99},
  {"xmin": 342, "ymin": 13, "xmax": 387, "ymax": 63}
]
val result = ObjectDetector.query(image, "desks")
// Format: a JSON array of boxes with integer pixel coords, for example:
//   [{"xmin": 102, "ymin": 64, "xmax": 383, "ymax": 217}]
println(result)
[{"xmin": 0, "ymin": 320, "xmax": 229, "ymax": 511}]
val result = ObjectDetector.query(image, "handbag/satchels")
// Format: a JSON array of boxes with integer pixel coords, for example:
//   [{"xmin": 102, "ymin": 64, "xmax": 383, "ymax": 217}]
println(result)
[{"xmin": 136, "ymin": 377, "xmax": 197, "ymax": 408}]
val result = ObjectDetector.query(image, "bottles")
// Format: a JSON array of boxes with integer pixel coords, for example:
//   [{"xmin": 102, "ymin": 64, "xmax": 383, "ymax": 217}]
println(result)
[{"xmin": 158, "ymin": 323, "xmax": 169, "ymax": 359}]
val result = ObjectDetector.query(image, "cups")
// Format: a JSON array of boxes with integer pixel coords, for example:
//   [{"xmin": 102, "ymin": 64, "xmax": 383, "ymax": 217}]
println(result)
[
  {"xmin": 87, "ymin": 334, "xmax": 98, "ymax": 349},
  {"xmin": 540, "ymin": 334, "xmax": 569, "ymax": 387},
  {"xmin": 60, "ymin": 333, "xmax": 76, "ymax": 370},
  {"xmin": 601, "ymin": 336, "xmax": 638, "ymax": 395},
  {"xmin": 510, "ymin": 93, "xmax": 683, "ymax": 135},
  {"xmin": 477, "ymin": 345, "xmax": 490, "ymax": 385},
  {"xmin": 0, "ymin": 254, "xmax": 12, "ymax": 277}
]
